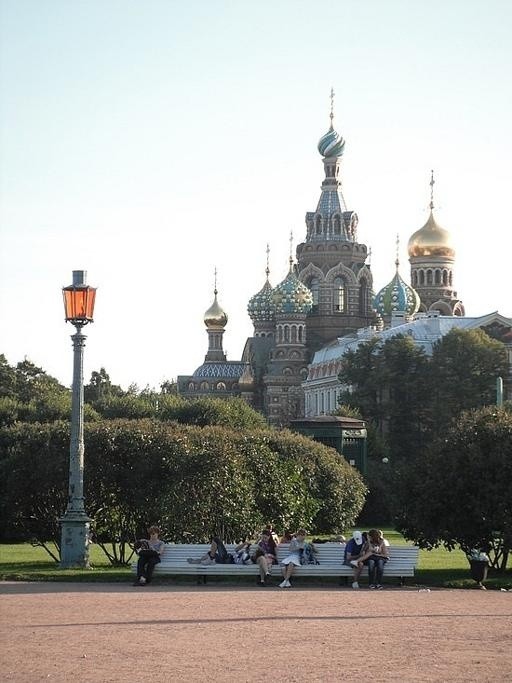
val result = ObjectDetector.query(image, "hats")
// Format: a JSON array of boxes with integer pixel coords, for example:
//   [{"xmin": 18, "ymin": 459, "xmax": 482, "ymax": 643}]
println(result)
[{"xmin": 353, "ymin": 530, "xmax": 362, "ymax": 546}]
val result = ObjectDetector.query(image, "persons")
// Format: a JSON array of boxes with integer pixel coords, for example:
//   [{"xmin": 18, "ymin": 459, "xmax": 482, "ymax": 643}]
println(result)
[
  {"xmin": 187, "ymin": 524, "xmax": 307, "ymax": 588},
  {"xmin": 343, "ymin": 529, "xmax": 390, "ymax": 589},
  {"xmin": 134, "ymin": 527, "xmax": 164, "ymax": 587}
]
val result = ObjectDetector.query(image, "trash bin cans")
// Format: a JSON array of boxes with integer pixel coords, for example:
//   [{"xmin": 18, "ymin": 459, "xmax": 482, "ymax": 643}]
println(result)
[{"xmin": 469, "ymin": 560, "xmax": 489, "ymax": 590}]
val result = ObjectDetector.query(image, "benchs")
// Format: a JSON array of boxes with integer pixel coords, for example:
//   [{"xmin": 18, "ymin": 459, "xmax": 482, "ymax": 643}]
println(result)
[{"xmin": 131, "ymin": 542, "xmax": 418, "ymax": 590}]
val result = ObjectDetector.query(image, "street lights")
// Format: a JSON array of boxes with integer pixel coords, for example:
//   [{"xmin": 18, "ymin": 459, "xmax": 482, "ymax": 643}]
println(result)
[{"xmin": 60, "ymin": 269, "xmax": 98, "ymax": 569}]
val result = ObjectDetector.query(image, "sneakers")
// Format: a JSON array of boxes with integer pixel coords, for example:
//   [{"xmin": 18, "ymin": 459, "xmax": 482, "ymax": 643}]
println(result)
[
  {"xmin": 349, "ymin": 559, "xmax": 360, "ymax": 569},
  {"xmin": 257, "ymin": 573, "xmax": 272, "ymax": 585},
  {"xmin": 200, "ymin": 556, "xmax": 216, "ymax": 566},
  {"xmin": 133, "ymin": 576, "xmax": 147, "ymax": 586},
  {"xmin": 368, "ymin": 583, "xmax": 384, "ymax": 590},
  {"xmin": 277, "ymin": 578, "xmax": 292, "ymax": 588},
  {"xmin": 351, "ymin": 581, "xmax": 360, "ymax": 589}
]
